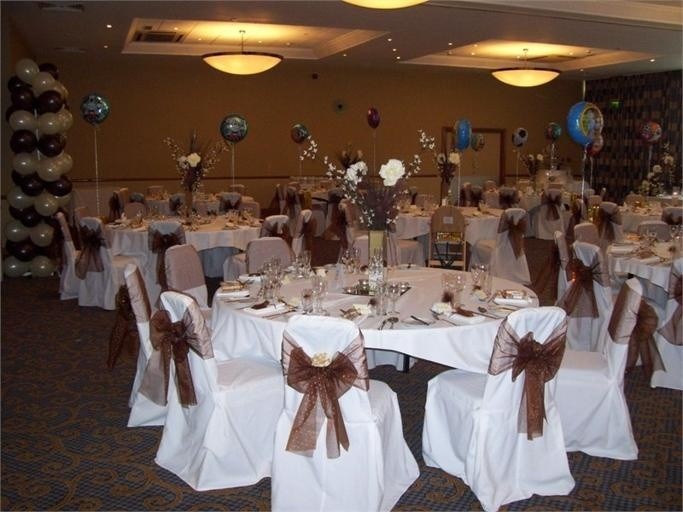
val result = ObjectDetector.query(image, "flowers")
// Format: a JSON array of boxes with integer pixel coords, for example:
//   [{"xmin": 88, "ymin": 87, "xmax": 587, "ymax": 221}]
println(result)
[
  {"xmin": 159, "ymin": 125, "xmax": 230, "ymax": 190},
  {"xmin": 295, "ymin": 126, "xmax": 461, "ymax": 231},
  {"xmin": 641, "ymin": 150, "xmax": 675, "ymax": 188},
  {"xmin": 517, "ymin": 146, "xmax": 543, "ymax": 179}
]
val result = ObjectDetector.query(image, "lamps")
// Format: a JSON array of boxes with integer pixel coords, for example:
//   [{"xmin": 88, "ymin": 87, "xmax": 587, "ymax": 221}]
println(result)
[
  {"xmin": 490, "ymin": 48, "xmax": 561, "ymax": 89},
  {"xmin": 200, "ymin": 30, "xmax": 283, "ymax": 76}
]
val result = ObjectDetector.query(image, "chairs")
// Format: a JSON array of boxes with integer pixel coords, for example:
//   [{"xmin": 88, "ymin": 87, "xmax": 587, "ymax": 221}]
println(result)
[
  {"xmin": 535, "ymin": 275, "xmax": 644, "ymax": 467},
  {"xmin": 58, "ymin": 175, "xmax": 680, "ymax": 420},
  {"xmin": 268, "ymin": 311, "xmax": 423, "ymax": 510},
  {"xmin": 147, "ymin": 287, "xmax": 278, "ymax": 496},
  {"xmin": 418, "ymin": 303, "xmax": 579, "ymax": 511}
]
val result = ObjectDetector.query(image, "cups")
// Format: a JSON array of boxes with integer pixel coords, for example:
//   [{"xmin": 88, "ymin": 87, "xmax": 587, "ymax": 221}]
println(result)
[
  {"xmin": 672, "ymin": 187, "xmax": 680, "ymax": 196},
  {"xmin": 133, "ymin": 207, "xmax": 255, "ymax": 228}
]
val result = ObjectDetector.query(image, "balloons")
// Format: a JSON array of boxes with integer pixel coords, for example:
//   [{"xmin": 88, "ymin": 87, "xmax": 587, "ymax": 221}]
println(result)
[
  {"xmin": 544, "ymin": 122, "xmax": 562, "ymax": 142},
  {"xmin": 582, "ymin": 135, "xmax": 604, "ymax": 157},
  {"xmin": 289, "ymin": 123, "xmax": 308, "ymax": 142},
  {"xmin": 80, "ymin": 94, "xmax": 109, "ymax": 124},
  {"xmin": 641, "ymin": 121, "xmax": 662, "ymax": 144},
  {"xmin": 219, "ymin": 115, "xmax": 247, "ymax": 141},
  {"xmin": 6, "ymin": 59, "xmax": 75, "ymax": 277},
  {"xmin": 568, "ymin": 101, "xmax": 603, "ymax": 147},
  {"xmin": 368, "ymin": 107, "xmax": 381, "ymax": 129},
  {"xmin": 511, "ymin": 127, "xmax": 528, "ymax": 147},
  {"xmin": 472, "ymin": 133, "xmax": 485, "ymax": 152},
  {"xmin": 453, "ymin": 118, "xmax": 472, "ymax": 151}
]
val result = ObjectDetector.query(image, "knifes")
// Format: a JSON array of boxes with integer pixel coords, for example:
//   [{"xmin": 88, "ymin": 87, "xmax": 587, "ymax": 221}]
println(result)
[
  {"xmin": 411, "ymin": 315, "xmax": 429, "ymax": 325},
  {"xmin": 378, "ymin": 318, "xmax": 388, "ymax": 332}
]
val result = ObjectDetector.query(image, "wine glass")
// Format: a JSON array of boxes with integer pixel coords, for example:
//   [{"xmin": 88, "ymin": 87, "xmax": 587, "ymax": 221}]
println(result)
[
  {"xmin": 257, "ymin": 247, "xmax": 493, "ymax": 316},
  {"xmin": 643, "ymin": 222, "xmax": 683, "ymax": 253}
]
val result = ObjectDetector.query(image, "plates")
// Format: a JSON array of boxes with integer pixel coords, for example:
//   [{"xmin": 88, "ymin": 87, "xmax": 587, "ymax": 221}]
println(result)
[{"xmin": 397, "ymin": 316, "xmax": 436, "ymax": 327}]
